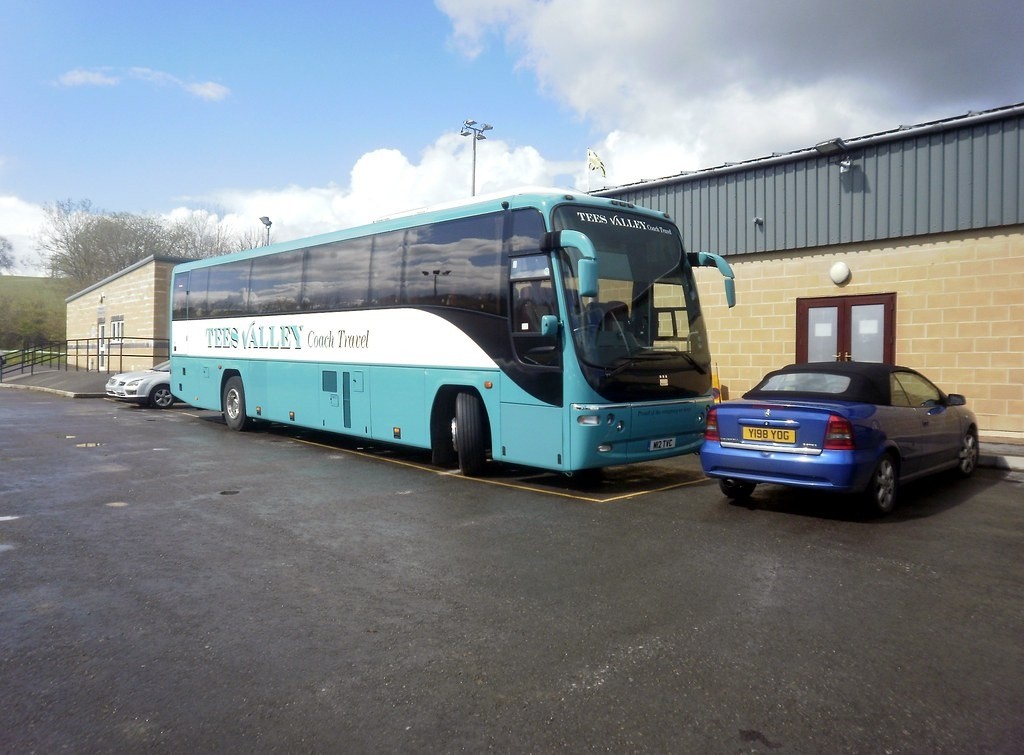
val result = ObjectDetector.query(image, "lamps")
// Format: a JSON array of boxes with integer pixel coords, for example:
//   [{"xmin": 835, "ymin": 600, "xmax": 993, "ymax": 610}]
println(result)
[{"xmin": 816, "ymin": 136, "xmax": 854, "ymax": 174}]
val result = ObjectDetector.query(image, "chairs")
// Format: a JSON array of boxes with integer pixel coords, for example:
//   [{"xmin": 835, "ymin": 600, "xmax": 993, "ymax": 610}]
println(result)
[{"xmin": 447, "ymin": 281, "xmax": 635, "ymax": 332}]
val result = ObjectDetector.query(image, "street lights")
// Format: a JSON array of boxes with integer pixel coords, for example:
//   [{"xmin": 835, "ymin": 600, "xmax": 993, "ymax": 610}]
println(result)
[
  {"xmin": 259, "ymin": 217, "xmax": 272, "ymax": 245},
  {"xmin": 459, "ymin": 119, "xmax": 494, "ymax": 197}
]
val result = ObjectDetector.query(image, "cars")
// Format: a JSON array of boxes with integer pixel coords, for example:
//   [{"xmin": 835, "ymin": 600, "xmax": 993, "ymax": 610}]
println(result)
[
  {"xmin": 104, "ymin": 359, "xmax": 171, "ymax": 404},
  {"xmin": 116, "ymin": 361, "xmax": 187, "ymax": 409},
  {"xmin": 701, "ymin": 361, "xmax": 980, "ymax": 517}
]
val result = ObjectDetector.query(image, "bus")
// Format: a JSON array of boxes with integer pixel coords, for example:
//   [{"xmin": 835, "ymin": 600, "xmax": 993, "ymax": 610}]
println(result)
[{"xmin": 168, "ymin": 190, "xmax": 736, "ymax": 480}]
